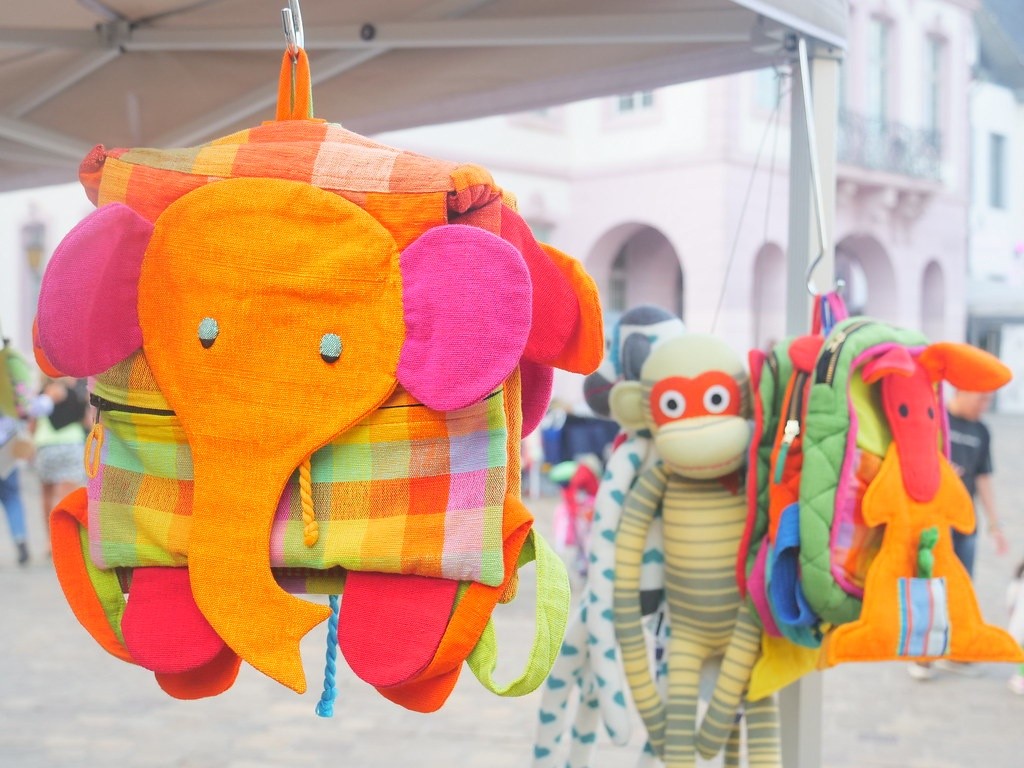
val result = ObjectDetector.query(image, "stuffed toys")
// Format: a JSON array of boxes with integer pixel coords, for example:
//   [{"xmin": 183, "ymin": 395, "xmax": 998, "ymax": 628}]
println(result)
[{"xmin": 534, "ymin": 317, "xmax": 781, "ymax": 768}]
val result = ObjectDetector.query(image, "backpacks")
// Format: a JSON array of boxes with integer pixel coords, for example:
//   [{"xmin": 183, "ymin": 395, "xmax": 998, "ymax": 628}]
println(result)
[{"xmin": 33, "ymin": 55, "xmax": 601, "ymax": 714}]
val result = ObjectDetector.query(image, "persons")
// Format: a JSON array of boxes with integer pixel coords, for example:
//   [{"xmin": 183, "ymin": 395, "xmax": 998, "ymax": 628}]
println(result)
[
  {"xmin": 0, "ymin": 321, "xmax": 101, "ymax": 563},
  {"xmin": 1002, "ymin": 560, "xmax": 1024, "ymax": 694},
  {"xmin": 907, "ymin": 386, "xmax": 1010, "ymax": 678}
]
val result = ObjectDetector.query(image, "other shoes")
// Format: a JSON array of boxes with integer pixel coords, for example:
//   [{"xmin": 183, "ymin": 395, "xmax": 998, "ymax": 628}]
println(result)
[
  {"xmin": 19, "ymin": 543, "xmax": 28, "ymax": 561},
  {"xmin": 934, "ymin": 659, "xmax": 972, "ymax": 674},
  {"xmin": 1008, "ymin": 675, "xmax": 1024, "ymax": 694},
  {"xmin": 907, "ymin": 661, "xmax": 935, "ymax": 679}
]
